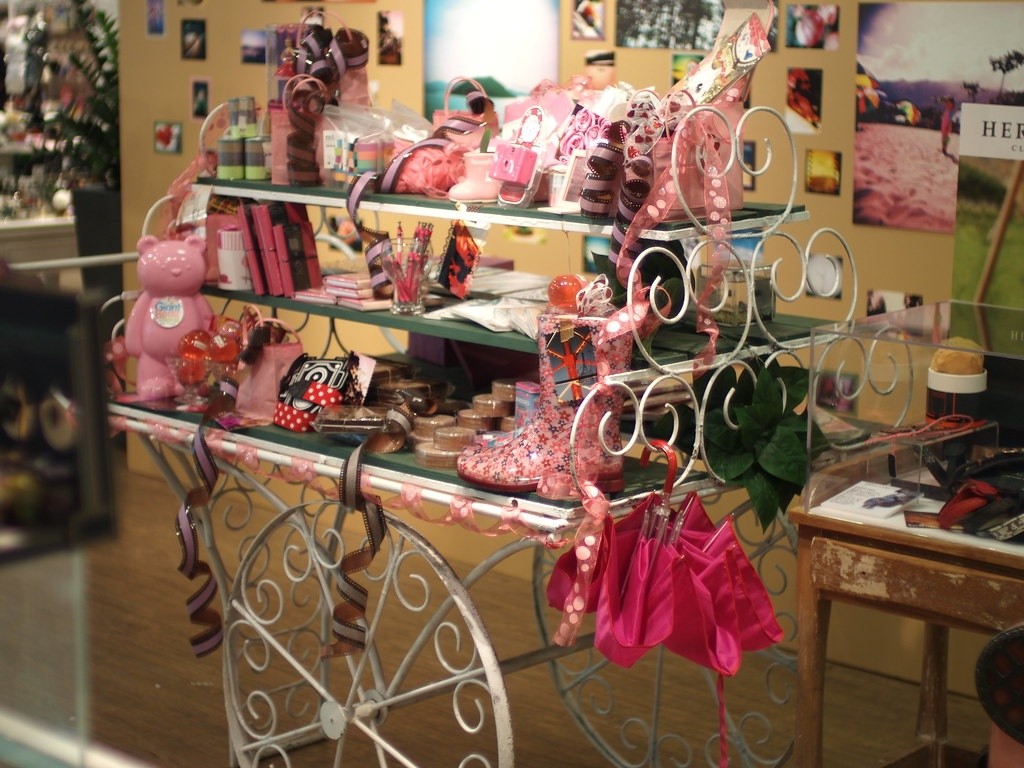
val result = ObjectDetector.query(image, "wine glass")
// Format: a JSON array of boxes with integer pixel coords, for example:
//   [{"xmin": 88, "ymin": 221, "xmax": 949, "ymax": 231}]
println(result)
[{"xmin": 170, "ymin": 359, "xmax": 246, "ymax": 403}]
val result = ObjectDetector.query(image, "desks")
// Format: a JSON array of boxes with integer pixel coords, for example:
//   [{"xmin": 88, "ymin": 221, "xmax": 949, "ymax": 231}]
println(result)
[{"xmin": 785, "ymin": 427, "xmax": 1024, "ymax": 768}]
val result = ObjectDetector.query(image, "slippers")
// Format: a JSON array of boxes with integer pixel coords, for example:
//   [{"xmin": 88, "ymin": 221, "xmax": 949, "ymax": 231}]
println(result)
[{"xmin": 491, "ymin": 106, "xmax": 553, "ymax": 208}]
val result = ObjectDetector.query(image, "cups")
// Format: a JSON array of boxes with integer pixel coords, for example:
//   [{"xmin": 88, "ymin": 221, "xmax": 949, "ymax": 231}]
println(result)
[
  {"xmin": 245, "ymin": 136, "xmax": 272, "ymax": 179},
  {"xmin": 217, "ymin": 139, "xmax": 243, "ymax": 179},
  {"xmin": 926, "ymin": 366, "xmax": 989, "ymax": 427},
  {"xmin": 381, "ymin": 238, "xmax": 434, "ymax": 315}
]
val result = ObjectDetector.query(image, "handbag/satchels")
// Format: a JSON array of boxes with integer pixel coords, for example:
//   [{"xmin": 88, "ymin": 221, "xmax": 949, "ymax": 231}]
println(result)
[
  {"xmin": 235, "ymin": 305, "xmax": 304, "ymax": 421},
  {"xmin": 629, "ymin": 88, "xmax": 714, "ymax": 219},
  {"xmin": 269, "ymin": 74, "xmax": 337, "ymax": 187},
  {"xmin": 289, "ymin": 9, "xmax": 371, "ymax": 110},
  {"xmin": 274, "ymin": 351, "xmax": 363, "ymax": 433},
  {"xmin": 435, "ymin": 77, "xmax": 489, "ymax": 136}
]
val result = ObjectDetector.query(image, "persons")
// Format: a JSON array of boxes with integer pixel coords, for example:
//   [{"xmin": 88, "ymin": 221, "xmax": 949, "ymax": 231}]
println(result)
[{"xmin": 938, "ymin": 96, "xmax": 959, "ymax": 158}]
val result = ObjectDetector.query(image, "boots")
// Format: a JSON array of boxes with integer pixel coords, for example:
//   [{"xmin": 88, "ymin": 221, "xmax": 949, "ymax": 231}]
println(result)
[
  {"xmin": 449, "ymin": 152, "xmax": 500, "ymax": 203},
  {"xmin": 456, "ymin": 315, "xmax": 636, "ymax": 494}
]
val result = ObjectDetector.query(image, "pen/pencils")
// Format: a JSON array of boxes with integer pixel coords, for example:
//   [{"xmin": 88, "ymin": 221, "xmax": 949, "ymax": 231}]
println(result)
[{"xmin": 383, "ymin": 221, "xmax": 433, "ymax": 306}]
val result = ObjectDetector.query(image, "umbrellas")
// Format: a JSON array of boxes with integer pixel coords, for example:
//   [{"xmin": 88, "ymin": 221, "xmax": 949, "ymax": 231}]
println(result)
[
  {"xmin": 548, "ymin": 439, "xmax": 785, "ymax": 676},
  {"xmin": 856, "ymin": 62, "xmax": 921, "ymax": 126}
]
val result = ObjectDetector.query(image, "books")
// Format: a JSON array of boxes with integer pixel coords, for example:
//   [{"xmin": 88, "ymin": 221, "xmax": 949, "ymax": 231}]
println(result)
[
  {"xmin": 237, "ymin": 203, "xmax": 323, "ymax": 298},
  {"xmin": 292, "ymin": 273, "xmax": 393, "ymax": 311},
  {"xmin": 820, "ymin": 481, "xmax": 963, "ymax": 530}
]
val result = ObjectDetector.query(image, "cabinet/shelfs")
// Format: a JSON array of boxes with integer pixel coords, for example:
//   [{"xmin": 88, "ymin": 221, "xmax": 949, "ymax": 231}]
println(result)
[{"xmin": 110, "ymin": 172, "xmax": 881, "ymax": 534}]
